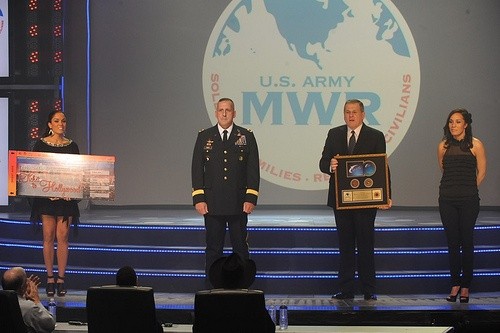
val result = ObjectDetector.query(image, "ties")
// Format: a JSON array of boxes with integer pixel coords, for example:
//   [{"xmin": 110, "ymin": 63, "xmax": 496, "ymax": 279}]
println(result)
[
  {"xmin": 223, "ymin": 130, "xmax": 228, "ymax": 144},
  {"xmin": 348, "ymin": 130, "xmax": 356, "ymax": 155}
]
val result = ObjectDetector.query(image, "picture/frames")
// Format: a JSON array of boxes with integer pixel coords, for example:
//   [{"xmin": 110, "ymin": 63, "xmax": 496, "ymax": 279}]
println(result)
[{"xmin": 334, "ymin": 154, "xmax": 390, "ymax": 210}]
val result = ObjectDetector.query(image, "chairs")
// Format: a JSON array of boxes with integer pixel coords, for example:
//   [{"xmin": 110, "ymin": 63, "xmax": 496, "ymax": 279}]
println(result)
[
  {"xmin": 0, "ymin": 290, "xmax": 27, "ymax": 333},
  {"xmin": 86, "ymin": 286, "xmax": 164, "ymax": 333},
  {"xmin": 192, "ymin": 290, "xmax": 276, "ymax": 333}
]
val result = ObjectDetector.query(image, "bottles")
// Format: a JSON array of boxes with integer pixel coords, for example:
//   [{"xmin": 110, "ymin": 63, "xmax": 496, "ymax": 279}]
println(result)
[
  {"xmin": 268, "ymin": 302, "xmax": 276, "ymax": 327},
  {"xmin": 280, "ymin": 302, "xmax": 288, "ymax": 330},
  {"xmin": 48, "ymin": 298, "xmax": 56, "ymax": 327}
]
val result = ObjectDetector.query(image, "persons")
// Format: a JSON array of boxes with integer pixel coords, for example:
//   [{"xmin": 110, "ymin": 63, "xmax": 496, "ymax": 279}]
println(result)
[
  {"xmin": 192, "ymin": 97, "xmax": 260, "ymax": 290},
  {"xmin": 438, "ymin": 108, "xmax": 487, "ymax": 302},
  {"xmin": 26, "ymin": 110, "xmax": 82, "ymax": 298},
  {"xmin": 0, "ymin": 267, "xmax": 55, "ymax": 333},
  {"xmin": 116, "ymin": 265, "xmax": 138, "ymax": 287},
  {"xmin": 319, "ymin": 99, "xmax": 392, "ymax": 301},
  {"xmin": 210, "ymin": 252, "xmax": 257, "ymax": 291}
]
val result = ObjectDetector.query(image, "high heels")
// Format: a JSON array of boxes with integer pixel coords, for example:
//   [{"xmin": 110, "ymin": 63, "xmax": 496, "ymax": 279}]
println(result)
[
  {"xmin": 446, "ymin": 287, "xmax": 460, "ymax": 302},
  {"xmin": 46, "ymin": 275, "xmax": 55, "ymax": 296},
  {"xmin": 459, "ymin": 288, "xmax": 469, "ymax": 303},
  {"xmin": 57, "ymin": 275, "xmax": 67, "ymax": 296}
]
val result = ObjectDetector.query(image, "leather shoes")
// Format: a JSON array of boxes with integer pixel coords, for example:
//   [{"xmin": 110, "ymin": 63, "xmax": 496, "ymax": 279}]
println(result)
[
  {"xmin": 363, "ymin": 294, "xmax": 377, "ymax": 300},
  {"xmin": 332, "ymin": 292, "xmax": 354, "ymax": 300}
]
